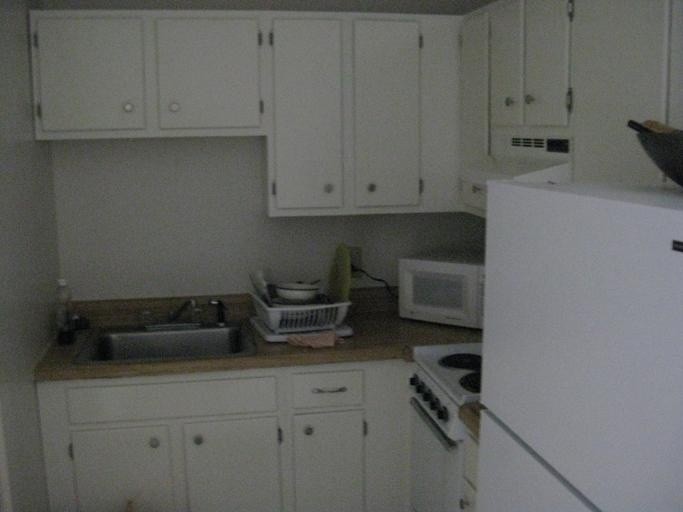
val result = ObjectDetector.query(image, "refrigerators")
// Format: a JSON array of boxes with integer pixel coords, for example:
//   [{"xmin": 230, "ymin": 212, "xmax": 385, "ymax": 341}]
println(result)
[{"xmin": 470, "ymin": 177, "xmax": 682, "ymax": 512}]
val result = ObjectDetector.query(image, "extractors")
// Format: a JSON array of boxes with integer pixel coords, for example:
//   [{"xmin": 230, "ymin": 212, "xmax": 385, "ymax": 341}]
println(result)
[{"xmin": 486, "ymin": 126, "xmax": 571, "ymax": 165}]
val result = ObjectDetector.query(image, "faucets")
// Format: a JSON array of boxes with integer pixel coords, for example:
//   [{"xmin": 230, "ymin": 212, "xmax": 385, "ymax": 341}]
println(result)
[
  {"xmin": 207, "ymin": 299, "xmax": 229, "ymax": 327},
  {"xmin": 167, "ymin": 297, "xmax": 198, "ymax": 323}
]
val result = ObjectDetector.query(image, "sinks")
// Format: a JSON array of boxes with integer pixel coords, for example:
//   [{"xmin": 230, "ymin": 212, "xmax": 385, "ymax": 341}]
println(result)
[{"xmin": 73, "ymin": 318, "xmax": 258, "ymax": 365}]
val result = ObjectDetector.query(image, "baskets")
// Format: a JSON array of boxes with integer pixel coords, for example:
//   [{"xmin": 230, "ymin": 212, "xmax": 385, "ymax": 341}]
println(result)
[{"xmin": 248, "ymin": 291, "xmax": 354, "ymax": 334}]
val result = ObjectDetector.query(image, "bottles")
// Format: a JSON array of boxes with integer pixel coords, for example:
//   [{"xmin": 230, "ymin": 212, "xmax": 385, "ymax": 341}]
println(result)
[{"xmin": 49, "ymin": 278, "xmax": 71, "ymax": 334}]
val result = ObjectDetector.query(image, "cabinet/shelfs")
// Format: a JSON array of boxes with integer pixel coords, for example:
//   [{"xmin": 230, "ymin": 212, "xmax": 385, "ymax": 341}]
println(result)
[
  {"xmin": 459, "ymin": 424, "xmax": 481, "ymax": 512},
  {"xmin": 34, "ymin": 369, "xmax": 285, "ymax": 512},
  {"xmin": 29, "ymin": 11, "xmax": 267, "ymax": 135},
  {"xmin": 460, "ymin": 2, "xmax": 488, "ymax": 214},
  {"xmin": 490, "ymin": 2, "xmax": 567, "ymax": 128},
  {"xmin": 289, "ymin": 361, "xmax": 368, "ymax": 510},
  {"xmin": 267, "ymin": 14, "xmax": 419, "ymax": 216},
  {"xmin": 570, "ymin": 3, "xmax": 666, "ymax": 183}
]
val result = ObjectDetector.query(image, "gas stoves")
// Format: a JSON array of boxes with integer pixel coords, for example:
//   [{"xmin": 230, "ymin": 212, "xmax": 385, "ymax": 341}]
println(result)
[{"xmin": 407, "ymin": 341, "xmax": 478, "ymax": 440}]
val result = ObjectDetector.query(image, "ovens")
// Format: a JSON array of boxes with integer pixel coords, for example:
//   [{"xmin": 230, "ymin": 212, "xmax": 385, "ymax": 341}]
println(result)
[{"xmin": 407, "ymin": 395, "xmax": 465, "ymax": 510}]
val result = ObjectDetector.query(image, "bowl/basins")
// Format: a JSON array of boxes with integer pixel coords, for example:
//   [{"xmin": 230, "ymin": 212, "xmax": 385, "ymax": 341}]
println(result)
[
  {"xmin": 635, "ymin": 132, "xmax": 681, "ymax": 188},
  {"xmin": 273, "ymin": 283, "xmax": 321, "ymax": 301}
]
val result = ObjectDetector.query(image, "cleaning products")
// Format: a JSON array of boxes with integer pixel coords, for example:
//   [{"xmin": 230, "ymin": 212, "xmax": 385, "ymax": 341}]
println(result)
[{"xmin": 54, "ymin": 278, "xmax": 75, "ymax": 347}]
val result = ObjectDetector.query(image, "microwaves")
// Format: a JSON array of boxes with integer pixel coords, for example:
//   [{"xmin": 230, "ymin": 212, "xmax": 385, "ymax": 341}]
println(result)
[{"xmin": 398, "ymin": 243, "xmax": 484, "ymax": 328}]
